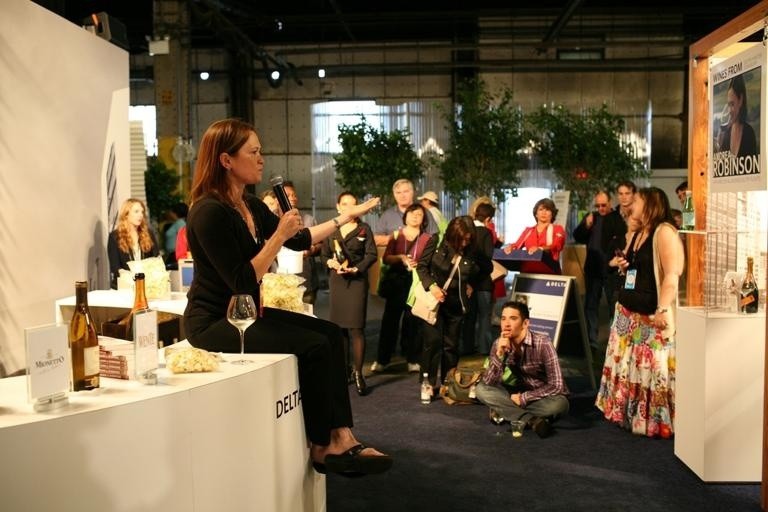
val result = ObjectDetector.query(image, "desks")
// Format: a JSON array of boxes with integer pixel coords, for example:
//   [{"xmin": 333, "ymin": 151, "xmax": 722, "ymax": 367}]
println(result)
[{"xmin": 0, "ymin": 290, "xmax": 327, "ymax": 512}]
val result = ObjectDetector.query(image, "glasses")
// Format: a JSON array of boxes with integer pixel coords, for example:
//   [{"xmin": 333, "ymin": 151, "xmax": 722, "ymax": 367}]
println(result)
[{"xmin": 595, "ymin": 199, "xmax": 609, "ymax": 210}]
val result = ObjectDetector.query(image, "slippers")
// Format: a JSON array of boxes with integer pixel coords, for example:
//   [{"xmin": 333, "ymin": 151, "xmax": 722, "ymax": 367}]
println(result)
[{"xmin": 311, "ymin": 441, "xmax": 391, "ymax": 475}]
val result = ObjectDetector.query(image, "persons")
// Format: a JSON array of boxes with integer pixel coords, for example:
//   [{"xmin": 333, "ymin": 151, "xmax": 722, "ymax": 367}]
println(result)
[
  {"xmin": 573, "ymin": 190, "xmax": 614, "ymax": 355},
  {"xmin": 474, "ymin": 301, "xmax": 569, "ymax": 438},
  {"xmin": 184, "ymin": 119, "xmax": 391, "ymax": 479},
  {"xmin": 716, "ymin": 77, "xmax": 757, "ymax": 175},
  {"xmin": 417, "ymin": 191, "xmax": 446, "ymax": 242},
  {"xmin": 597, "ymin": 186, "xmax": 683, "ymax": 436},
  {"xmin": 321, "ymin": 192, "xmax": 377, "ymax": 394},
  {"xmin": 464, "ymin": 203, "xmax": 493, "ymax": 355},
  {"xmin": 371, "ymin": 203, "xmax": 430, "ymax": 371},
  {"xmin": 260, "ymin": 190, "xmax": 279, "ymax": 215},
  {"xmin": 166, "ymin": 202, "xmax": 189, "ymax": 269},
  {"xmin": 468, "ymin": 198, "xmax": 505, "ymax": 356},
  {"xmin": 506, "ymin": 198, "xmax": 566, "ymax": 272},
  {"xmin": 603, "ymin": 181, "xmax": 635, "ymax": 316},
  {"xmin": 276, "ymin": 179, "xmax": 319, "ymax": 278},
  {"xmin": 108, "ymin": 198, "xmax": 166, "ymax": 291},
  {"xmin": 374, "ymin": 178, "xmax": 439, "ymax": 244},
  {"xmin": 417, "ymin": 216, "xmax": 493, "ymax": 405}
]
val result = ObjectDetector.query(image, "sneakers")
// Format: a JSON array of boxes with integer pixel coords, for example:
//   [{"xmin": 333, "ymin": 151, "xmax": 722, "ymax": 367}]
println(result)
[
  {"xmin": 419, "ymin": 379, "xmax": 433, "ymax": 405},
  {"xmin": 371, "ymin": 361, "xmax": 386, "ymax": 373},
  {"xmin": 409, "ymin": 363, "xmax": 422, "ymax": 373}
]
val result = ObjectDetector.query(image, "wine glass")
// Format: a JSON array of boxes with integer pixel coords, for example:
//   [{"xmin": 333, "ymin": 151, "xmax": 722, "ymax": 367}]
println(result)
[
  {"xmin": 735, "ymin": 272, "xmax": 756, "ymax": 316},
  {"xmin": 226, "ymin": 294, "xmax": 258, "ymax": 363}
]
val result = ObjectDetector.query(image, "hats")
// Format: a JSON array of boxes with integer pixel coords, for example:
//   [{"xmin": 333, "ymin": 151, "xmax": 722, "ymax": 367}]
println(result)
[{"xmin": 416, "ymin": 191, "xmax": 440, "ymax": 204}]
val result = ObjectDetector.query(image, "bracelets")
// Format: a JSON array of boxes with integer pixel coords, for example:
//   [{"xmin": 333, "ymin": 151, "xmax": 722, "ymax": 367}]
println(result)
[{"xmin": 333, "ymin": 219, "xmax": 340, "ymax": 233}]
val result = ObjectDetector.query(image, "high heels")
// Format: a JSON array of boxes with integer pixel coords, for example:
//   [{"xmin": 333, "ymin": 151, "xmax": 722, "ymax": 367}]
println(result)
[{"xmin": 354, "ymin": 369, "xmax": 367, "ymax": 395}]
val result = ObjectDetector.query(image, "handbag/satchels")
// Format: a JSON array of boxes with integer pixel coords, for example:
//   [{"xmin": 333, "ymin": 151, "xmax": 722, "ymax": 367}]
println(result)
[
  {"xmin": 377, "ymin": 230, "xmax": 402, "ymax": 297},
  {"xmin": 438, "ymin": 367, "xmax": 483, "ymax": 404},
  {"xmin": 653, "ymin": 223, "xmax": 694, "ymax": 338},
  {"xmin": 411, "ymin": 255, "xmax": 462, "ymax": 326}
]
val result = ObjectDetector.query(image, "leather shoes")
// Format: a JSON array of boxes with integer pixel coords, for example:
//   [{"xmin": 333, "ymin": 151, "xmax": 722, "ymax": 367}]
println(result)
[
  {"xmin": 490, "ymin": 407, "xmax": 505, "ymax": 423},
  {"xmin": 529, "ymin": 419, "xmax": 550, "ymax": 437}
]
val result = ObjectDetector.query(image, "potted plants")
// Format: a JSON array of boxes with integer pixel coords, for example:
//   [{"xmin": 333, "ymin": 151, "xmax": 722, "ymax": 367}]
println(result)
[
  {"xmin": 335, "ymin": 121, "xmax": 424, "ymax": 296},
  {"xmin": 541, "ymin": 106, "xmax": 641, "ymax": 297}
]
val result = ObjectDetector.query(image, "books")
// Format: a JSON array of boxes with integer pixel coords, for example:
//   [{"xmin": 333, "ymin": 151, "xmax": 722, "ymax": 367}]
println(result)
[{"xmin": 97, "ymin": 336, "xmax": 135, "ymax": 380}]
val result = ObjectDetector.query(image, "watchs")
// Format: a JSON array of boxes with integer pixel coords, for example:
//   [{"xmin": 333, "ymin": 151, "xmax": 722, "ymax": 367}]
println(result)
[{"xmin": 656, "ymin": 307, "xmax": 668, "ymax": 314}]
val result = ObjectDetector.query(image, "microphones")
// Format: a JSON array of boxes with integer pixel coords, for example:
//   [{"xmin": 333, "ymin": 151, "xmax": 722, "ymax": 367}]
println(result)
[{"xmin": 268, "ymin": 172, "xmax": 304, "ymax": 238}]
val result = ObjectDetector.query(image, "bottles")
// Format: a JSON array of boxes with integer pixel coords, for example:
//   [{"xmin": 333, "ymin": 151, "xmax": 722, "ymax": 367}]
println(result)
[
  {"xmin": 331, "ymin": 237, "xmax": 351, "ymax": 268},
  {"xmin": 68, "ymin": 282, "xmax": 99, "ymax": 392},
  {"xmin": 613, "ymin": 247, "xmax": 638, "ymax": 275},
  {"xmin": 125, "ymin": 274, "xmax": 151, "ymax": 341},
  {"xmin": 681, "ymin": 190, "xmax": 696, "ymax": 230},
  {"xmin": 742, "ymin": 258, "xmax": 759, "ymax": 314},
  {"xmin": 421, "ymin": 372, "xmax": 432, "ymax": 405}
]
